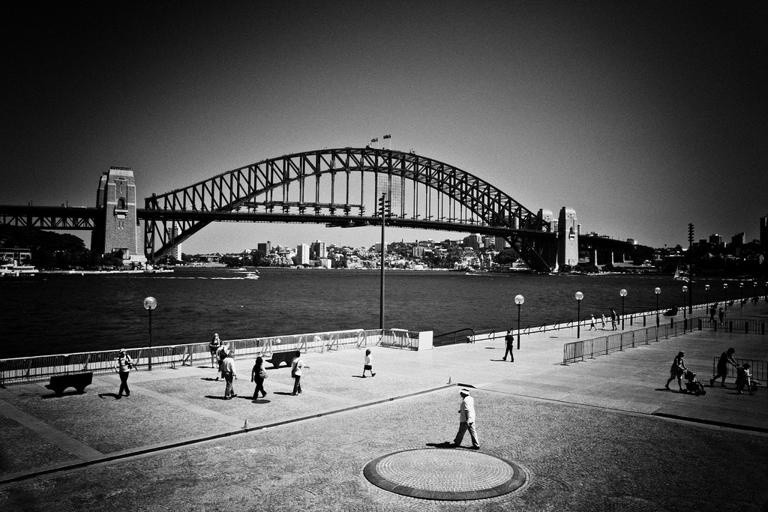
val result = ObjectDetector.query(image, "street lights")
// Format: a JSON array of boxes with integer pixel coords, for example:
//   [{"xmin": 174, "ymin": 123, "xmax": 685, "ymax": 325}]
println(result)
[
  {"xmin": 705, "ymin": 284, "xmax": 710, "ymax": 316},
  {"xmin": 377, "ymin": 191, "xmax": 392, "ymax": 329},
  {"xmin": 654, "ymin": 287, "xmax": 661, "ymax": 316},
  {"xmin": 723, "ymin": 282, "xmax": 728, "ymax": 311},
  {"xmin": 618, "ymin": 288, "xmax": 628, "ymax": 330},
  {"xmin": 144, "ymin": 295, "xmax": 158, "ymax": 370},
  {"xmin": 739, "ymin": 281, "xmax": 768, "ymax": 308},
  {"xmin": 687, "ymin": 221, "xmax": 693, "ymax": 314},
  {"xmin": 575, "ymin": 291, "xmax": 585, "ymax": 340},
  {"xmin": 515, "ymin": 293, "xmax": 525, "ymax": 350},
  {"xmin": 682, "ymin": 286, "xmax": 688, "ymax": 319}
]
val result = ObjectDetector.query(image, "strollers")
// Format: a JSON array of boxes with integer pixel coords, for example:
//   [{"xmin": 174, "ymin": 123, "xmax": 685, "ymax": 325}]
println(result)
[{"xmin": 681, "ymin": 368, "xmax": 706, "ymax": 397}]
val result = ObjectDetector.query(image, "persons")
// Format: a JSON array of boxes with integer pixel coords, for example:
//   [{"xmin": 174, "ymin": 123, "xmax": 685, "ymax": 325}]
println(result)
[
  {"xmin": 727, "ymin": 294, "xmax": 761, "ymax": 307},
  {"xmin": 664, "ymin": 350, "xmax": 688, "ymax": 391},
  {"xmin": 215, "ymin": 341, "xmax": 230, "ymax": 381},
  {"xmin": 113, "ymin": 348, "xmax": 133, "ymax": 399},
  {"xmin": 210, "ymin": 332, "xmax": 221, "ymax": 368},
  {"xmin": 709, "ymin": 305, "xmax": 716, "ymax": 321},
  {"xmin": 610, "ymin": 308, "xmax": 618, "ymax": 330},
  {"xmin": 710, "ymin": 347, "xmax": 738, "ymax": 386},
  {"xmin": 718, "ymin": 308, "xmax": 724, "ymax": 325},
  {"xmin": 588, "ymin": 314, "xmax": 598, "ymax": 331},
  {"xmin": 691, "ymin": 371, "xmax": 705, "ymax": 392},
  {"xmin": 250, "ymin": 356, "xmax": 268, "ymax": 401},
  {"xmin": 453, "ymin": 389, "xmax": 481, "ymax": 450},
  {"xmin": 600, "ymin": 311, "xmax": 606, "ymax": 329},
  {"xmin": 291, "ymin": 351, "xmax": 310, "ymax": 394},
  {"xmin": 743, "ymin": 363, "xmax": 759, "ymax": 390},
  {"xmin": 222, "ymin": 352, "xmax": 238, "ymax": 400},
  {"xmin": 362, "ymin": 348, "xmax": 376, "ymax": 378},
  {"xmin": 503, "ymin": 329, "xmax": 514, "ymax": 361}
]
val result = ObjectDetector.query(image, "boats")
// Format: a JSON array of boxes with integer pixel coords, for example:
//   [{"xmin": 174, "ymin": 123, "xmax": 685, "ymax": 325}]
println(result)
[{"xmin": 175, "ymin": 261, "xmax": 263, "ymax": 280}]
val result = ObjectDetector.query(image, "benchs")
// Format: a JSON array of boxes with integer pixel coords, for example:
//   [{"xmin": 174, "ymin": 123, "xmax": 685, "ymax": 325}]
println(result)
[
  {"xmin": 45, "ymin": 372, "xmax": 92, "ymax": 394},
  {"xmin": 265, "ymin": 350, "xmax": 300, "ymax": 367}
]
